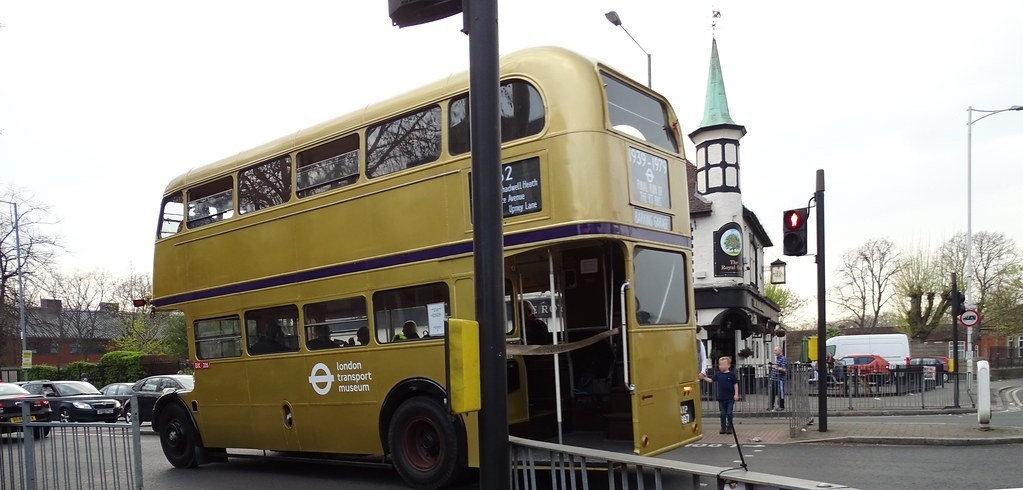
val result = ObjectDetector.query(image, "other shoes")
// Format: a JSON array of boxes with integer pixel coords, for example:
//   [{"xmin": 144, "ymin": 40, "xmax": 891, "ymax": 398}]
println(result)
[
  {"xmin": 720, "ymin": 425, "xmax": 726, "ymax": 434},
  {"xmin": 776, "ymin": 407, "xmax": 784, "ymax": 411},
  {"xmin": 727, "ymin": 426, "xmax": 732, "ymax": 434},
  {"xmin": 767, "ymin": 406, "xmax": 774, "ymax": 410}
]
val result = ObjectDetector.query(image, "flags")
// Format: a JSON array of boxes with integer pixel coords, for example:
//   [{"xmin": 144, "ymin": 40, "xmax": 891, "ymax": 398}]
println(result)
[{"xmin": 713, "ymin": 10, "xmax": 721, "ymax": 18}]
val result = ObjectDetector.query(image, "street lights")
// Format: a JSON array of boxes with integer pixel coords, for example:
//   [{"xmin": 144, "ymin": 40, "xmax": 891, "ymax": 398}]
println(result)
[
  {"xmin": 966, "ymin": 105, "xmax": 1023, "ymax": 392},
  {"xmin": 605, "ymin": 11, "xmax": 652, "ymax": 88},
  {"xmin": 387, "ymin": 1, "xmax": 513, "ymax": 490}
]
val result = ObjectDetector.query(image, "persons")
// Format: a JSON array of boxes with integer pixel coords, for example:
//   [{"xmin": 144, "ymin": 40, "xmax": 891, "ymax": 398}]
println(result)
[
  {"xmin": 80, "ymin": 373, "xmax": 90, "ymax": 383},
  {"xmin": 306, "ymin": 314, "xmax": 342, "ymax": 351},
  {"xmin": 249, "ymin": 319, "xmax": 294, "ymax": 355},
  {"xmin": 178, "ymin": 369, "xmax": 186, "ymax": 375},
  {"xmin": 767, "ymin": 346, "xmax": 788, "ymax": 411},
  {"xmin": 696, "ymin": 324, "xmax": 706, "ymax": 380},
  {"xmin": 826, "ymin": 352, "xmax": 843, "ymax": 372},
  {"xmin": 177, "ymin": 202, "xmax": 218, "ymax": 233},
  {"xmin": 392, "ymin": 319, "xmax": 420, "ymax": 341},
  {"xmin": 344, "ymin": 327, "xmax": 370, "ymax": 346},
  {"xmin": 700, "ymin": 356, "xmax": 739, "ymax": 434},
  {"xmin": 518, "ymin": 300, "xmax": 549, "ymax": 345}
]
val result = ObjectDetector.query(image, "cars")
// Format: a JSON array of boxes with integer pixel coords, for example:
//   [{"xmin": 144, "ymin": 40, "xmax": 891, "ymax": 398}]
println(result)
[
  {"xmin": 910, "ymin": 357, "xmax": 949, "ymax": 385},
  {"xmin": 100, "ymin": 383, "xmax": 156, "ymax": 416},
  {"xmin": 21, "ymin": 380, "xmax": 124, "ymax": 424},
  {"xmin": 0, "ymin": 381, "xmax": 53, "ymax": 438},
  {"xmin": 834, "ymin": 354, "xmax": 892, "ymax": 386},
  {"xmin": 121, "ymin": 374, "xmax": 195, "ymax": 426}
]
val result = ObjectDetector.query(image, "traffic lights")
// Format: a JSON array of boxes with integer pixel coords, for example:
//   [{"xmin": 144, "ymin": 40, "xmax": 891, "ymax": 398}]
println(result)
[
  {"xmin": 958, "ymin": 292, "xmax": 966, "ymax": 316},
  {"xmin": 783, "ymin": 208, "xmax": 807, "ymax": 256}
]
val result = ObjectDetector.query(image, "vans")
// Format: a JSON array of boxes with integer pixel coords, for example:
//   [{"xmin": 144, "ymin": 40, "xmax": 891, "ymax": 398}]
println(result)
[{"xmin": 826, "ymin": 334, "xmax": 909, "ymax": 377}]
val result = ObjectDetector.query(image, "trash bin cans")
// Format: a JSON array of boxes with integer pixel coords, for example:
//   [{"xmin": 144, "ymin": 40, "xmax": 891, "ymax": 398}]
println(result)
[{"xmin": 910, "ymin": 357, "xmax": 943, "ymax": 386}]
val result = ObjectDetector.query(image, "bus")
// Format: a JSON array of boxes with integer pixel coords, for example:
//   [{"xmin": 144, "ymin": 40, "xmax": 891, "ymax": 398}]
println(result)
[{"xmin": 153, "ymin": 45, "xmax": 704, "ymax": 490}]
